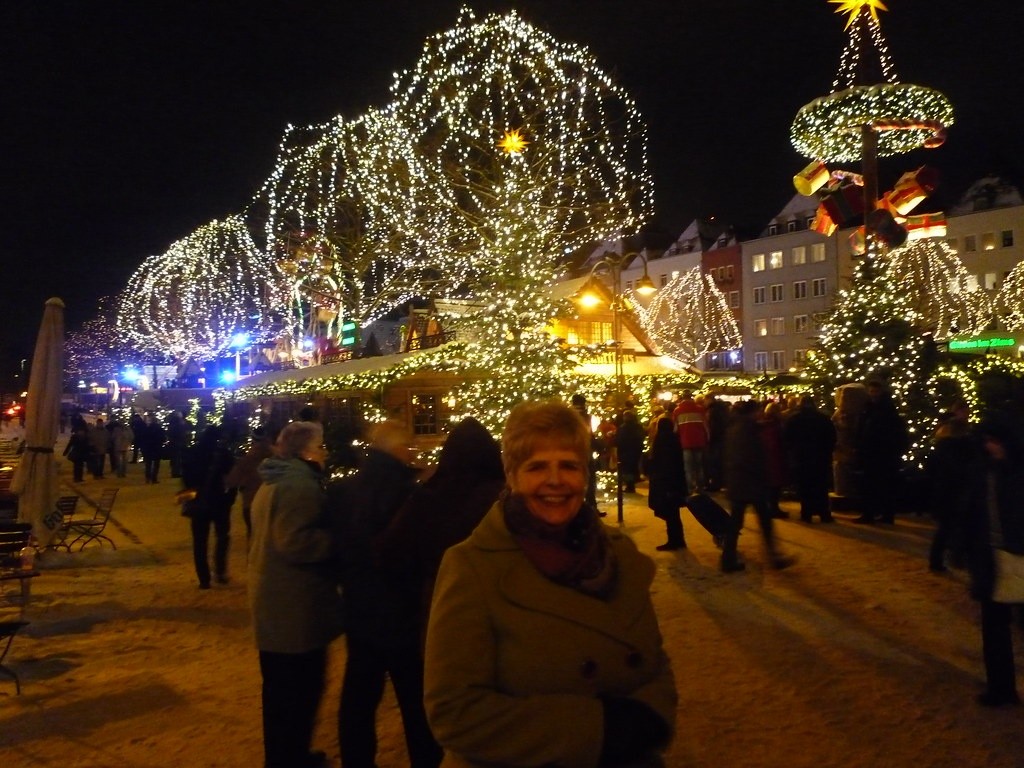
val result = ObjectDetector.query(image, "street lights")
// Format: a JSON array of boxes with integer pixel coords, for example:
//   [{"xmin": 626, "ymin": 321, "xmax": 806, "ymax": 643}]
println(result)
[{"xmin": 573, "ymin": 251, "xmax": 657, "ymax": 522}]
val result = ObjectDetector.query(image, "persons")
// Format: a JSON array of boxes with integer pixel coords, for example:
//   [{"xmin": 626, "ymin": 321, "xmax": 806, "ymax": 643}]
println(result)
[
  {"xmin": 63, "ymin": 411, "xmax": 273, "ymax": 485},
  {"xmin": 248, "ymin": 422, "xmax": 331, "ymax": 767},
  {"xmin": 970, "ymin": 370, "xmax": 1024, "ymax": 705},
  {"xmin": 331, "ymin": 422, "xmax": 432, "ymax": 766},
  {"xmin": 422, "ymin": 402, "xmax": 679, "ymax": 768},
  {"xmin": 608, "ymin": 382, "xmax": 1023, "ymax": 567},
  {"xmin": 371, "ymin": 417, "xmax": 504, "ymax": 572},
  {"xmin": 181, "ymin": 432, "xmax": 239, "ymax": 589},
  {"xmin": 572, "ymin": 394, "xmax": 606, "ymax": 517}
]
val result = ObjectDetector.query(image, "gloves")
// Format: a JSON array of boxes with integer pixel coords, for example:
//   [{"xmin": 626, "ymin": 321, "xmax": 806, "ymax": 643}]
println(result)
[{"xmin": 603, "ymin": 698, "xmax": 672, "ymax": 768}]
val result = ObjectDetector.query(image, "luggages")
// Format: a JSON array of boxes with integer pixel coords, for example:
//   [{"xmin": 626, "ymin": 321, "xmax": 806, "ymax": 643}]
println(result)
[{"xmin": 687, "ymin": 492, "xmax": 731, "ymax": 546}]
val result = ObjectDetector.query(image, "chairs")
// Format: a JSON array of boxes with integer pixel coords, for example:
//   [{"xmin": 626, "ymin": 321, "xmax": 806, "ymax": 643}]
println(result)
[
  {"xmin": 0, "ymin": 620, "xmax": 31, "ymax": 696},
  {"xmin": 0, "ymin": 452, "xmax": 42, "ymax": 598},
  {"xmin": 41, "ymin": 494, "xmax": 77, "ymax": 552},
  {"xmin": 63, "ymin": 487, "xmax": 119, "ymax": 554}
]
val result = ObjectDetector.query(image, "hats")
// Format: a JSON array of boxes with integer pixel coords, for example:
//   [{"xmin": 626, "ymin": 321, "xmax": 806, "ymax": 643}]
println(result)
[
  {"xmin": 129, "ymin": 408, "xmax": 138, "ymax": 413},
  {"xmin": 251, "ymin": 426, "xmax": 269, "ymax": 439},
  {"xmin": 116, "ymin": 410, "xmax": 125, "ymax": 413},
  {"xmin": 734, "ymin": 399, "xmax": 758, "ymax": 415},
  {"xmin": 143, "ymin": 411, "xmax": 158, "ymax": 416},
  {"xmin": 682, "ymin": 389, "xmax": 696, "ymax": 396}
]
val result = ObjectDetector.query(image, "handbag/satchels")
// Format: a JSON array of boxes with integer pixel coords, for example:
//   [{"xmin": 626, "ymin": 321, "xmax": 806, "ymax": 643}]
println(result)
[{"xmin": 173, "ymin": 479, "xmax": 197, "ymax": 505}]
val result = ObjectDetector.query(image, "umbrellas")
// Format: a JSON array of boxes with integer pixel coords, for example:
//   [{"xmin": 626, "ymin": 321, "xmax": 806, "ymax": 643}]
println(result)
[{"xmin": 10, "ymin": 298, "xmax": 70, "ymax": 540}]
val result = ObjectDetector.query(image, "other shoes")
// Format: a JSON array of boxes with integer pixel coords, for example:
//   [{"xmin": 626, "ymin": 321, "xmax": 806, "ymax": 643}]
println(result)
[
  {"xmin": 198, "ymin": 583, "xmax": 211, "ymax": 589},
  {"xmin": 622, "ymin": 487, "xmax": 636, "ymax": 493},
  {"xmin": 214, "ymin": 574, "xmax": 232, "ymax": 584},
  {"xmin": 656, "ymin": 540, "xmax": 686, "ymax": 552},
  {"xmin": 770, "ymin": 506, "xmax": 789, "ymax": 519},
  {"xmin": 719, "ymin": 558, "xmax": 746, "ymax": 573},
  {"xmin": 972, "ymin": 689, "xmax": 1019, "ymax": 709},
  {"xmin": 151, "ymin": 479, "xmax": 160, "ymax": 484},
  {"xmin": 772, "ymin": 557, "xmax": 793, "ymax": 570},
  {"xmin": 127, "ymin": 460, "xmax": 137, "ymax": 464}
]
